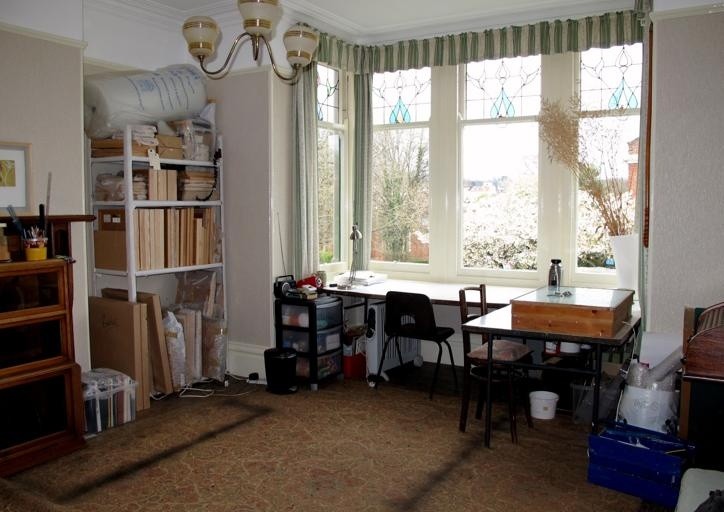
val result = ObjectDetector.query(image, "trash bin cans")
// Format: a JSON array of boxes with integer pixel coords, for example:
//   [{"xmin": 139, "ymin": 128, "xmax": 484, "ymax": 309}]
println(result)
[{"xmin": 264, "ymin": 347, "xmax": 299, "ymax": 395}]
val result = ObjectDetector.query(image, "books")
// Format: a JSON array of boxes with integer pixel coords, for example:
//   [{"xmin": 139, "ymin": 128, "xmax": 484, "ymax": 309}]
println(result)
[{"xmin": 82, "ymin": 163, "xmax": 223, "ymax": 434}]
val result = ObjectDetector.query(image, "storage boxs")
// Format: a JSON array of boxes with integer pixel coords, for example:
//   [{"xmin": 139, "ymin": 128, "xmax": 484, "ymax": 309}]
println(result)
[
  {"xmin": 80, "ymin": 368, "xmax": 139, "ymax": 436},
  {"xmin": 588, "ymin": 419, "xmax": 695, "ymax": 509}
]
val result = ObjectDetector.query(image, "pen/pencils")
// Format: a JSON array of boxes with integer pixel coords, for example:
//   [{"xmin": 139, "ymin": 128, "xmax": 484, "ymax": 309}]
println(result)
[{"xmin": 22, "ymin": 225, "xmax": 45, "ymax": 248}]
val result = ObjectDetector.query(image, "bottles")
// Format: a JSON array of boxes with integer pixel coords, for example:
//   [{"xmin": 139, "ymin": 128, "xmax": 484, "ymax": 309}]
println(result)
[
  {"xmin": 546, "ymin": 259, "xmax": 564, "ymax": 297},
  {"xmin": 629, "ymin": 362, "xmax": 650, "ymax": 388}
]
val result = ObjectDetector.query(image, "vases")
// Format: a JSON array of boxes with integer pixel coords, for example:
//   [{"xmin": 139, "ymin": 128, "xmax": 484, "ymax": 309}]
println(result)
[{"xmin": 609, "ymin": 232, "xmax": 642, "ymax": 311}]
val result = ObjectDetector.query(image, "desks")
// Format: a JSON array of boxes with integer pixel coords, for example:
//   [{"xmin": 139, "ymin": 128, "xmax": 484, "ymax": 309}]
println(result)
[
  {"xmin": 320, "ymin": 279, "xmax": 538, "ymax": 340},
  {"xmin": 461, "ymin": 300, "xmax": 642, "ymax": 448}
]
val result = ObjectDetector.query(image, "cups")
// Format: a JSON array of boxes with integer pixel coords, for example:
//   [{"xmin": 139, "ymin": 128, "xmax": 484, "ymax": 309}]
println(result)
[
  {"xmin": 337, "ymin": 275, "xmax": 348, "ymax": 289},
  {"xmin": 311, "ymin": 271, "xmax": 327, "ymax": 287},
  {"xmin": 23, "ymin": 238, "xmax": 48, "ymax": 261}
]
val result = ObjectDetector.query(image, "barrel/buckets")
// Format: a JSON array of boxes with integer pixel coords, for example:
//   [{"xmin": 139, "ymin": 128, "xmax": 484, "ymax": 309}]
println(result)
[
  {"xmin": 545, "ymin": 341, "xmax": 561, "ymax": 356},
  {"xmin": 560, "ymin": 341, "xmax": 581, "ymax": 354},
  {"xmin": 529, "ymin": 391, "xmax": 560, "ymax": 420}
]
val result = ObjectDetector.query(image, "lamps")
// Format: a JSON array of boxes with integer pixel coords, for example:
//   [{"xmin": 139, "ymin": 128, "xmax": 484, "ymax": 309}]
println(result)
[
  {"xmin": 182, "ymin": 0, "xmax": 318, "ymax": 80},
  {"xmin": 348, "ymin": 222, "xmax": 363, "ymax": 284}
]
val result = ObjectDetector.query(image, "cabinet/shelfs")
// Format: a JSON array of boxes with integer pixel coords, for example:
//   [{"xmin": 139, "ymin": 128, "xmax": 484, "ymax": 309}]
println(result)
[
  {"xmin": 0, "ymin": 215, "xmax": 98, "ymax": 479},
  {"xmin": 87, "ymin": 130, "xmax": 227, "ymax": 398},
  {"xmin": 275, "ymin": 295, "xmax": 343, "ymax": 391}
]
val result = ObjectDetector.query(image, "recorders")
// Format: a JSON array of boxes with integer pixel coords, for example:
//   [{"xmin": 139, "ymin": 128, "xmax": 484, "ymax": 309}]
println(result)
[{"xmin": 274, "ymin": 274, "xmax": 296, "ymax": 298}]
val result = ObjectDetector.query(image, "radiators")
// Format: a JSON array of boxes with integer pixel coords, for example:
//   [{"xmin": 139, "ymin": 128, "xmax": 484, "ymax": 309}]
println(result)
[{"xmin": 366, "ymin": 301, "xmax": 423, "ymax": 383}]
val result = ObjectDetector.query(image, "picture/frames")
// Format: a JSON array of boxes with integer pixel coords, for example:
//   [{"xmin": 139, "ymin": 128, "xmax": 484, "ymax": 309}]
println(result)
[{"xmin": 0, "ymin": 141, "xmax": 32, "ymax": 218}]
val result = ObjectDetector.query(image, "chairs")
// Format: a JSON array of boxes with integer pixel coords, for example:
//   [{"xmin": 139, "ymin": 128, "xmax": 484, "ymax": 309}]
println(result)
[
  {"xmin": 459, "ymin": 284, "xmax": 535, "ymax": 443},
  {"xmin": 374, "ymin": 291, "xmax": 457, "ymax": 400}
]
